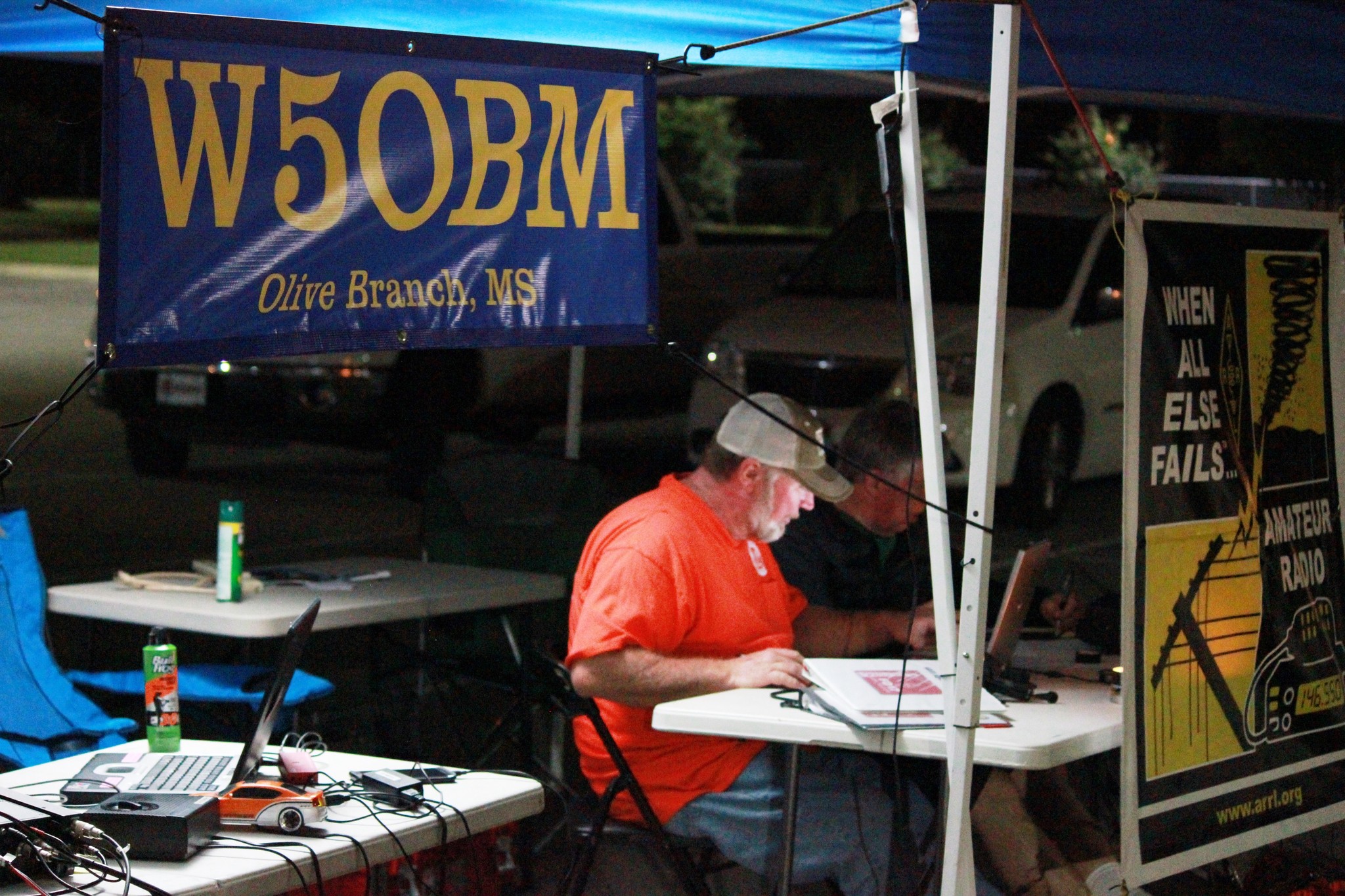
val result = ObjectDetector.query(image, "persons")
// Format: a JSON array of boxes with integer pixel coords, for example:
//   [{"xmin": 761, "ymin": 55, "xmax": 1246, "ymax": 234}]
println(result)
[
  {"xmin": 770, "ymin": 398, "xmax": 1152, "ymax": 896},
  {"xmin": 565, "ymin": 394, "xmax": 1000, "ymax": 896}
]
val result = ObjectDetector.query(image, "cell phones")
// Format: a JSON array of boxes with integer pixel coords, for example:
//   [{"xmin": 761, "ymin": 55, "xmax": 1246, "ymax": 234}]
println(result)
[{"xmin": 351, "ymin": 767, "xmax": 456, "ymax": 784}]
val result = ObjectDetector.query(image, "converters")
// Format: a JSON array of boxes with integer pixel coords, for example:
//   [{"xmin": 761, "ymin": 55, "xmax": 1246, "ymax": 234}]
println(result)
[{"xmin": 362, "ymin": 769, "xmax": 424, "ymax": 808}]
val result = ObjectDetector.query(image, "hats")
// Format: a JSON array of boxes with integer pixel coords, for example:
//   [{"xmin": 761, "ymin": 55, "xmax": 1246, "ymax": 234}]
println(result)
[{"xmin": 716, "ymin": 392, "xmax": 854, "ymax": 503}]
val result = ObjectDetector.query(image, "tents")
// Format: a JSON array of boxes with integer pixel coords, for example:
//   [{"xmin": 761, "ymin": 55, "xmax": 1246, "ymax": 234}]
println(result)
[{"xmin": 4, "ymin": 2, "xmax": 1344, "ymax": 895}]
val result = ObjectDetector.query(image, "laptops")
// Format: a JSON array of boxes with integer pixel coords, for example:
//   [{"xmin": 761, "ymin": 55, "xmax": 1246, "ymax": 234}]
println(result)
[
  {"xmin": 60, "ymin": 597, "xmax": 320, "ymax": 805},
  {"xmin": 986, "ymin": 541, "xmax": 1050, "ymax": 668}
]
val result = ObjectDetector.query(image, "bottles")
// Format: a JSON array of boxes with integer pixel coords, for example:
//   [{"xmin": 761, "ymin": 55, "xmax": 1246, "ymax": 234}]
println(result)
[
  {"xmin": 215, "ymin": 500, "xmax": 245, "ymax": 603},
  {"xmin": 142, "ymin": 625, "xmax": 181, "ymax": 753}
]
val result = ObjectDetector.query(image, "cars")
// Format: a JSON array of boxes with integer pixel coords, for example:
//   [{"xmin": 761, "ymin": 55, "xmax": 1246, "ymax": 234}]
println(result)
[{"xmin": 684, "ymin": 189, "xmax": 1129, "ymax": 532}]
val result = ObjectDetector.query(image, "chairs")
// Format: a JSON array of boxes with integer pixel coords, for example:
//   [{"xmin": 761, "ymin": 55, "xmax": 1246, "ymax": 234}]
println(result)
[{"xmin": 0, "ymin": 509, "xmax": 341, "ymax": 768}]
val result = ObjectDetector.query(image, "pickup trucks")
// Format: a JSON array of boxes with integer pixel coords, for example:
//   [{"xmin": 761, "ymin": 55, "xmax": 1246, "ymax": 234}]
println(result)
[{"xmin": 79, "ymin": 148, "xmax": 827, "ymax": 500}]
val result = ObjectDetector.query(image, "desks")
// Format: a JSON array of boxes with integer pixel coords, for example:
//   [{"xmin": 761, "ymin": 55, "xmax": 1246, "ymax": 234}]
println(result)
[
  {"xmin": 651, "ymin": 658, "xmax": 1122, "ymax": 896},
  {"xmin": 48, "ymin": 556, "xmax": 566, "ymax": 699},
  {"xmin": 0, "ymin": 738, "xmax": 545, "ymax": 896}
]
